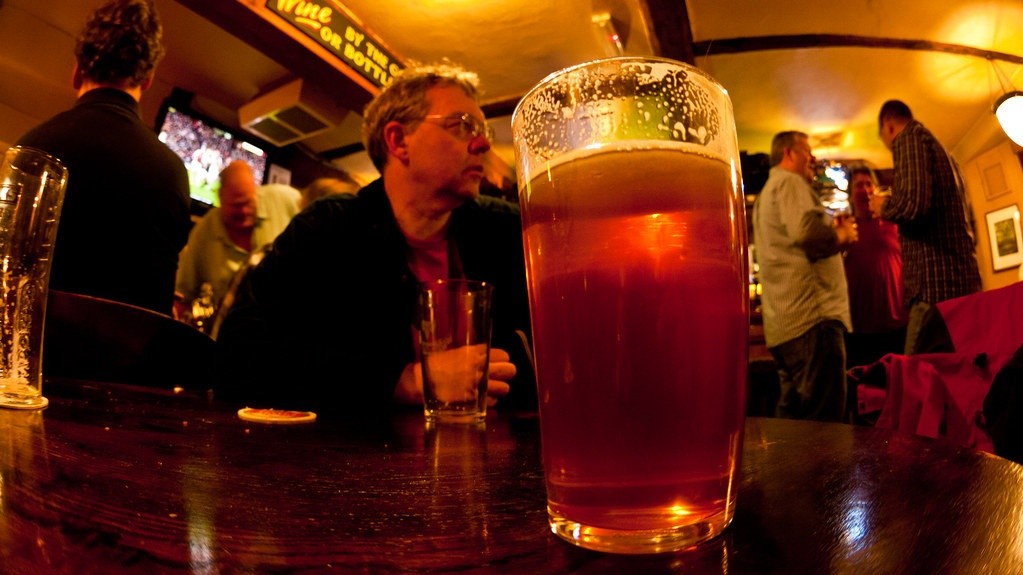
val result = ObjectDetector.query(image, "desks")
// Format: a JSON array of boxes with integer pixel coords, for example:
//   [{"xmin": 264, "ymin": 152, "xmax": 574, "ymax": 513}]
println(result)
[{"xmin": 0, "ymin": 390, "xmax": 1023, "ymax": 575}]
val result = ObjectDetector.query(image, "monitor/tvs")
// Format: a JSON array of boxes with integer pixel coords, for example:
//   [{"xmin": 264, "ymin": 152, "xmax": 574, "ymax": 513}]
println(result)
[{"xmin": 151, "ymin": 95, "xmax": 276, "ymax": 218}]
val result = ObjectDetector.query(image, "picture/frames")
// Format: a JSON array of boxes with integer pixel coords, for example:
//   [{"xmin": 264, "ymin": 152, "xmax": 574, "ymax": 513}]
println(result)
[{"xmin": 984, "ymin": 202, "xmax": 1023, "ymax": 272}]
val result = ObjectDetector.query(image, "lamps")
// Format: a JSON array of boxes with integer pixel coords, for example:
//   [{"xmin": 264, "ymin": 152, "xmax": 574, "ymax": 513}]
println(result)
[{"xmin": 985, "ymin": 54, "xmax": 1023, "ymax": 147}]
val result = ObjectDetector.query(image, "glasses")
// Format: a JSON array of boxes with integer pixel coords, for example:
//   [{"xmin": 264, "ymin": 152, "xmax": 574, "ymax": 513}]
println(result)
[{"xmin": 426, "ymin": 112, "xmax": 495, "ymax": 144}]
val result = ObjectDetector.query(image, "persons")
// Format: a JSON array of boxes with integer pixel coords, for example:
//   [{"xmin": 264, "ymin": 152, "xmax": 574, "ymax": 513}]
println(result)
[
  {"xmin": 868, "ymin": 98, "xmax": 983, "ymax": 356},
  {"xmin": 10, "ymin": 0, "xmax": 190, "ymax": 391},
  {"xmin": 298, "ymin": 179, "xmax": 359, "ymax": 214},
  {"xmin": 172, "ymin": 160, "xmax": 304, "ymax": 324},
  {"xmin": 839, "ymin": 163, "xmax": 907, "ymax": 368},
  {"xmin": 752, "ymin": 130, "xmax": 858, "ymax": 420},
  {"xmin": 210, "ymin": 64, "xmax": 541, "ymax": 417}
]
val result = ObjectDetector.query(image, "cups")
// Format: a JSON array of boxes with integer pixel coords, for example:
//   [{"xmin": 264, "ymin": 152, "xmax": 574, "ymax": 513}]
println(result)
[
  {"xmin": 873, "ymin": 186, "xmax": 892, "ymax": 197},
  {"xmin": 833, "ymin": 209, "xmax": 849, "ymax": 226},
  {"xmin": 412, "ymin": 278, "xmax": 495, "ymax": 424},
  {"xmin": 0, "ymin": 146, "xmax": 69, "ymax": 409},
  {"xmin": 509, "ymin": 56, "xmax": 749, "ymax": 553}
]
